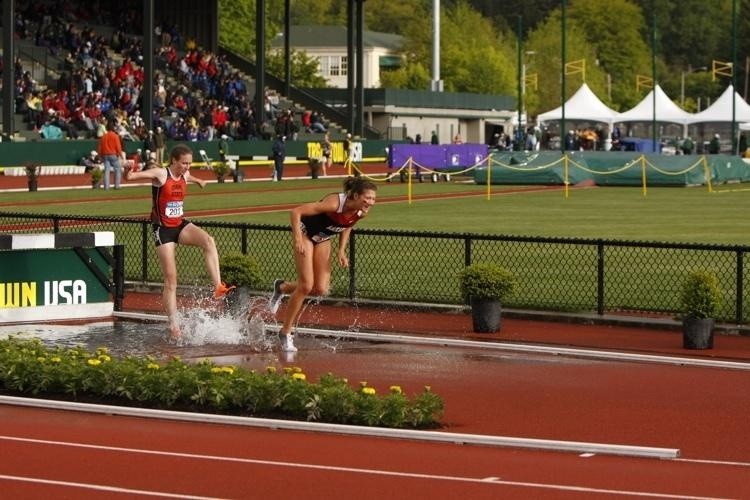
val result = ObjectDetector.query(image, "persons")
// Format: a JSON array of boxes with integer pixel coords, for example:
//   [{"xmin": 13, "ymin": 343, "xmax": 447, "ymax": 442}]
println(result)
[
  {"xmin": 415, "ymin": 131, "xmax": 438, "ymax": 145},
  {"xmin": 268, "ymin": 175, "xmax": 378, "ymax": 353},
  {"xmin": 1, "ymin": 1, "xmax": 354, "ymax": 192},
  {"xmin": 488, "ymin": 126, "xmax": 551, "ymax": 150},
  {"xmin": 564, "ymin": 126, "xmax": 621, "ymax": 151},
  {"xmin": 121, "ymin": 144, "xmax": 237, "ymax": 341},
  {"xmin": 671, "ymin": 133, "xmax": 748, "ymax": 157}
]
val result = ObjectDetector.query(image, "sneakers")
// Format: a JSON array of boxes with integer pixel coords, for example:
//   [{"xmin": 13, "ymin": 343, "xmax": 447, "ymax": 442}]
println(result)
[
  {"xmin": 212, "ymin": 282, "xmax": 237, "ymax": 300},
  {"xmin": 269, "ymin": 278, "xmax": 285, "ymax": 315},
  {"xmin": 278, "ymin": 327, "xmax": 299, "ymax": 353}
]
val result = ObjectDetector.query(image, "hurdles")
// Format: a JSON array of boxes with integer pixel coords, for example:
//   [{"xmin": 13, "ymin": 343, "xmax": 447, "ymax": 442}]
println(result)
[{"xmin": 0, "ymin": 231, "xmax": 125, "ymax": 322}]
